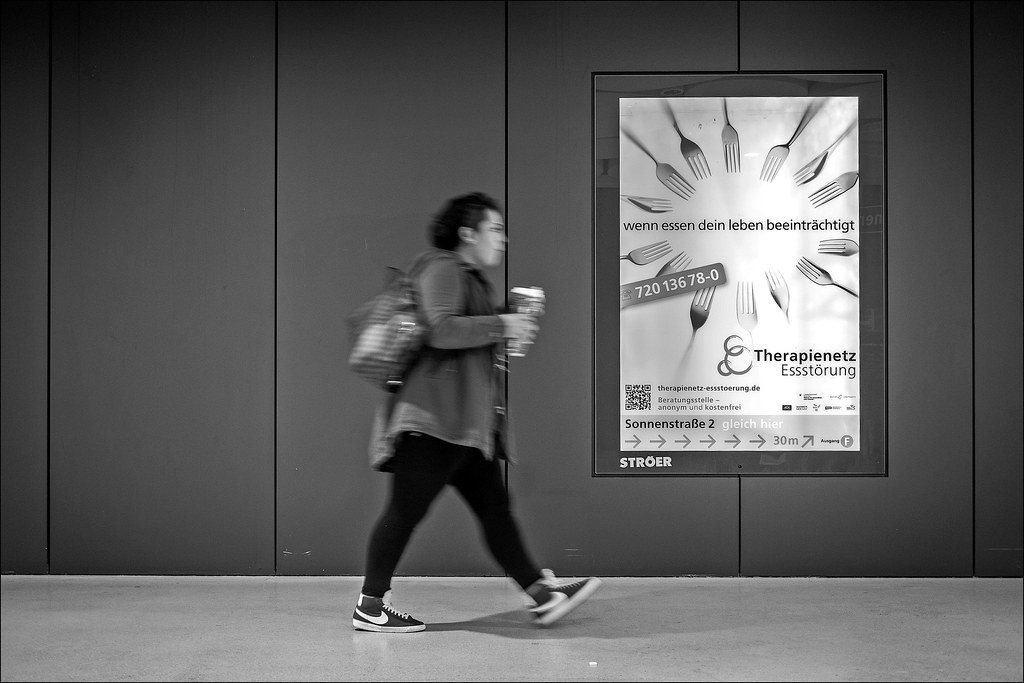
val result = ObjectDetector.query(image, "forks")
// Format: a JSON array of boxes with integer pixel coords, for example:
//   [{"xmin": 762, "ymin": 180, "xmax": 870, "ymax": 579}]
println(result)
[
  {"xmin": 808, "ymin": 171, "xmax": 859, "ymax": 208},
  {"xmin": 765, "ymin": 269, "xmax": 790, "ymax": 328},
  {"xmin": 621, "ymin": 194, "xmax": 674, "ymax": 213},
  {"xmin": 737, "ymin": 281, "xmax": 759, "ymax": 345},
  {"xmin": 622, "ymin": 125, "xmax": 697, "ymax": 201},
  {"xmin": 659, "ymin": 99, "xmax": 712, "ymax": 180},
  {"xmin": 760, "ymin": 97, "xmax": 828, "ymax": 183},
  {"xmin": 817, "ymin": 238, "xmax": 859, "ymax": 257},
  {"xmin": 796, "ymin": 255, "xmax": 859, "ymax": 299},
  {"xmin": 792, "ymin": 119, "xmax": 856, "ymax": 187},
  {"xmin": 620, "ymin": 240, "xmax": 673, "ymax": 266},
  {"xmin": 721, "ymin": 98, "xmax": 741, "ymax": 173},
  {"xmin": 654, "ymin": 251, "xmax": 693, "ymax": 277},
  {"xmin": 683, "ymin": 286, "xmax": 717, "ymax": 355}
]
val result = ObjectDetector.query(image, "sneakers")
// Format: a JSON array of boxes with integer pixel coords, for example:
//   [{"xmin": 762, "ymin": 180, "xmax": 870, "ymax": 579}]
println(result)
[
  {"xmin": 528, "ymin": 568, "xmax": 602, "ymax": 629},
  {"xmin": 352, "ymin": 589, "xmax": 427, "ymax": 634}
]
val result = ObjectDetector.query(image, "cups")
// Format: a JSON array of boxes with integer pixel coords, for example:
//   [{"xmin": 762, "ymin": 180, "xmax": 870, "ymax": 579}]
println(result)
[{"xmin": 503, "ymin": 285, "xmax": 546, "ymax": 358}]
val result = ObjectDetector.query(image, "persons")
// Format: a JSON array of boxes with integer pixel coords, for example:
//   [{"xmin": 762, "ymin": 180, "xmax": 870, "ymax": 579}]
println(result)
[{"xmin": 353, "ymin": 193, "xmax": 600, "ymax": 632}]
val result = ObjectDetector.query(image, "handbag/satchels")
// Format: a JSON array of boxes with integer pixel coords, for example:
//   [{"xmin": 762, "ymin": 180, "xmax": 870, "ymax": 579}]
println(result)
[{"xmin": 344, "ymin": 248, "xmax": 472, "ymax": 395}]
